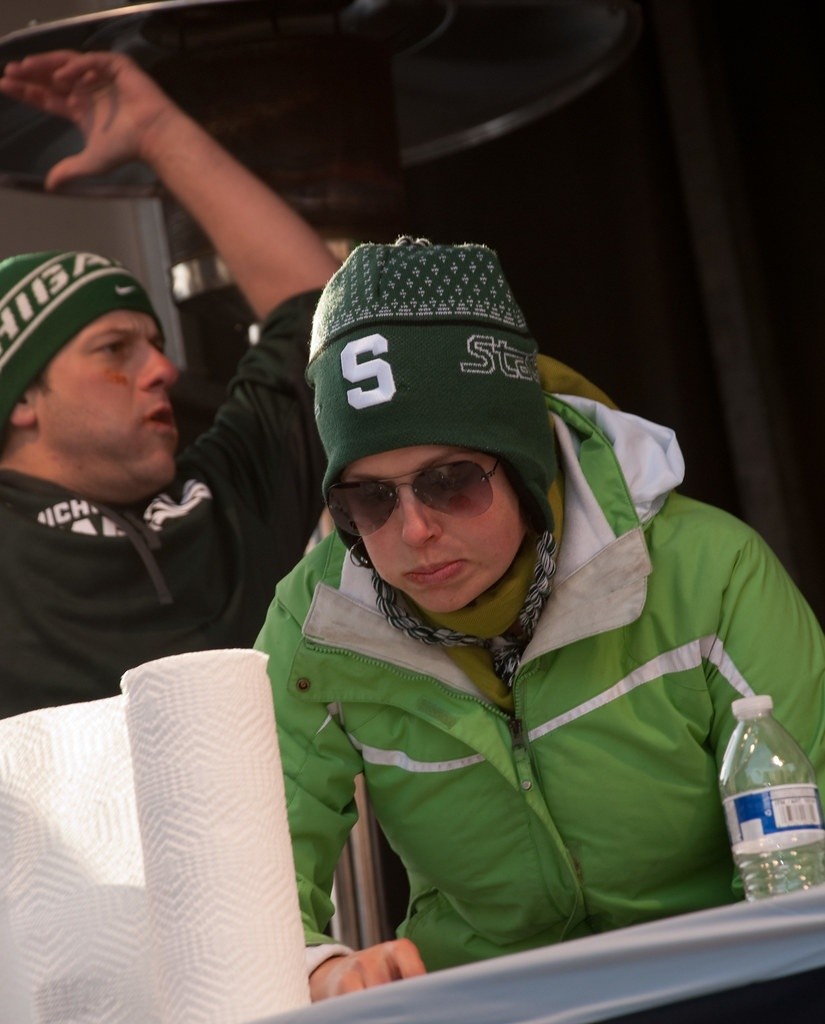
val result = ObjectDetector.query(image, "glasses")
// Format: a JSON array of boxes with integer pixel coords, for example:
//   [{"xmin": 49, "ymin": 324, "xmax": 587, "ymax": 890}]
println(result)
[{"xmin": 326, "ymin": 456, "xmax": 506, "ymax": 535}]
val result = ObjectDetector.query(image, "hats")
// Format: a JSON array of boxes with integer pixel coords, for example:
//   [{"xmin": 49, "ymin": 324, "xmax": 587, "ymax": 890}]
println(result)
[
  {"xmin": 0, "ymin": 250, "xmax": 164, "ymax": 427},
  {"xmin": 306, "ymin": 234, "xmax": 554, "ymax": 555}
]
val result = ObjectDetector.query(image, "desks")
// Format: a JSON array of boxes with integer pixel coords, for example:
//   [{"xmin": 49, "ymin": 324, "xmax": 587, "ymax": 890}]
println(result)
[{"xmin": 257, "ymin": 884, "xmax": 825, "ymax": 1024}]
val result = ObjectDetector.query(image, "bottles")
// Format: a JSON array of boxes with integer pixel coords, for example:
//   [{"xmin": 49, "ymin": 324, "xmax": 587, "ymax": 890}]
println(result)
[{"xmin": 718, "ymin": 695, "xmax": 825, "ymax": 903}]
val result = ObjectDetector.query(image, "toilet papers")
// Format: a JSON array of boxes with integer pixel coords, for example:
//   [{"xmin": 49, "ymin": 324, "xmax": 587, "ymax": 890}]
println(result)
[{"xmin": 0, "ymin": 648, "xmax": 314, "ymax": 1023}]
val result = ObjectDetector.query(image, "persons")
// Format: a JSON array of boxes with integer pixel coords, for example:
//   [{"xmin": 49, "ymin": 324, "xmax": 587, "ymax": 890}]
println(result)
[
  {"xmin": 251, "ymin": 236, "xmax": 825, "ymax": 999},
  {"xmin": 0, "ymin": 50, "xmax": 343, "ymax": 722}
]
